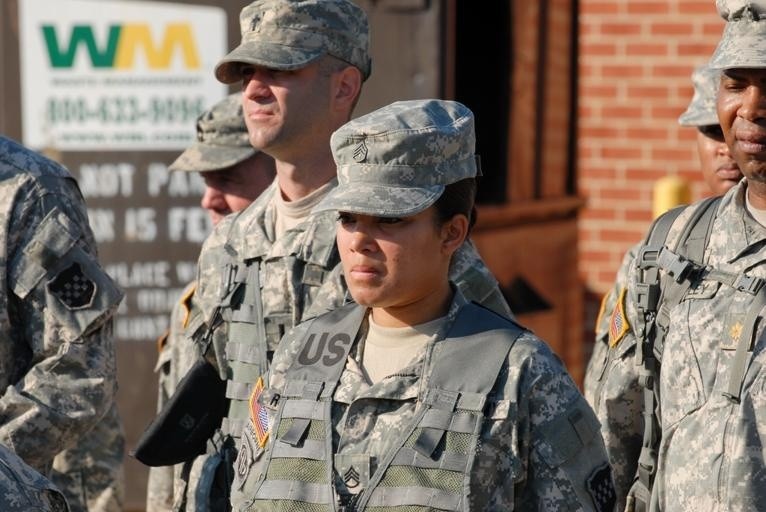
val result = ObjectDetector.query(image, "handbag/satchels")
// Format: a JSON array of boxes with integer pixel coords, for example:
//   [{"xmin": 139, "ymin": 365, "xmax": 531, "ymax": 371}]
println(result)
[{"xmin": 133, "ymin": 355, "xmax": 231, "ymax": 467}]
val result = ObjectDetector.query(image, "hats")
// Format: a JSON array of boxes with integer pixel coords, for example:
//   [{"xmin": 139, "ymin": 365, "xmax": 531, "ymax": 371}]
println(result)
[
  {"xmin": 678, "ymin": 63, "xmax": 720, "ymax": 127},
  {"xmin": 215, "ymin": 0, "xmax": 371, "ymax": 84},
  {"xmin": 312, "ymin": 99, "xmax": 483, "ymax": 218},
  {"xmin": 167, "ymin": 91, "xmax": 257, "ymax": 172},
  {"xmin": 710, "ymin": 0, "xmax": 766, "ymax": 70}
]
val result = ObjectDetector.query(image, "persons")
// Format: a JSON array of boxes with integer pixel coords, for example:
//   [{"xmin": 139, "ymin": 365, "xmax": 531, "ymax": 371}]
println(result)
[
  {"xmin": 147, "ymin": 92, "xmax": 276, "ymax": 512},
  {"xmin": 594, "ymin": 0, "xmax": 765, "ymax": 512},
  {"xmin": 581, "ymin": 61, "xmax": 747, "ymax": 512},
  {"xmin": 0, "ymin": 131, "xmax": 126, "ymax": 512},
  {"xmin": 230, "ymin": 100, "xmax": 618, "ymax": 512},
  {"xmin": 182, "ymin": 3, "xmax": 521, "ymax": 512}
]
